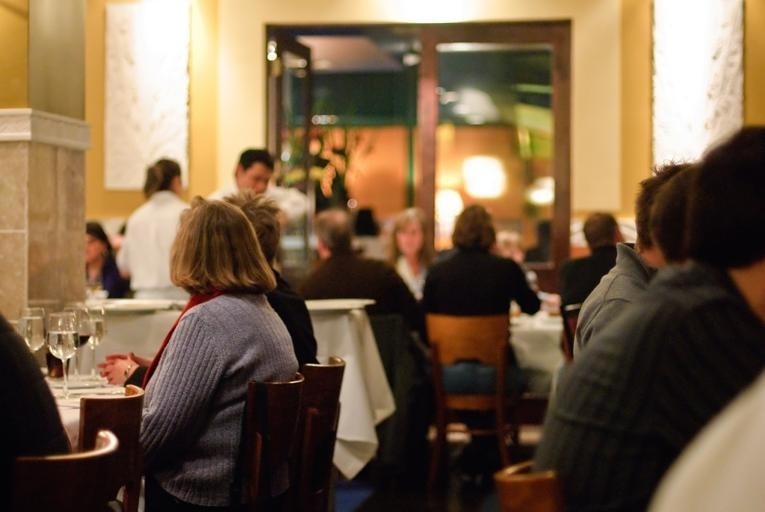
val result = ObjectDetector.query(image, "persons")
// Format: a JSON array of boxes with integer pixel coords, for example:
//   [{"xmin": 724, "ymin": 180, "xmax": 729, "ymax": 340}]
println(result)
[{"xmin": 1, "ymin": 122, "xmax": 765, "ymax": 511}]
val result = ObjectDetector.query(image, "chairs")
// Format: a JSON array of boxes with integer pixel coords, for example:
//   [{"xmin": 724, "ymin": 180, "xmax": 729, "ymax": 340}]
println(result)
[
  {"xmin": 494, "ymin": 459, "xmax": 566, "ymax": 512},
  {"xmin": 295, "ymin": 354, "xmax": 347, "ymax": 512},
  {"xmin": 75, "ymin": 383, "xmax": 146, "ymax": 512},
  {"xmin": 426, "ymin": 313, "xmax": 521, "ymax": 512},
  {"xmin": 1, "ymin": 429, "xmax": 124, "ymax": 512},
  {"xmin": 233, "ymin": 370, "xmax": 305, "ymax": 512}
]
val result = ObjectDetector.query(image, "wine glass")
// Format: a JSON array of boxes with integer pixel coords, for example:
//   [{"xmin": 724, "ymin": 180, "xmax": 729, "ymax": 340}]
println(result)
[
  {"xmin": 86, "ymin": 306, "xmax": 106, "ymax": 382},
  {"xmin": 63, "ymin": 307, "xmax": 92, "ymax": 379},
  {"xmin": 19, "ymin": 306, "xmax": 46, "ymax": 331},
  {"xmin": 18, "ymin": 317, "xmax": 46, "ymax": 353},
  {"xmin": 47, "ymin": 312, "xmax": 80, "ymax": 399}
]
